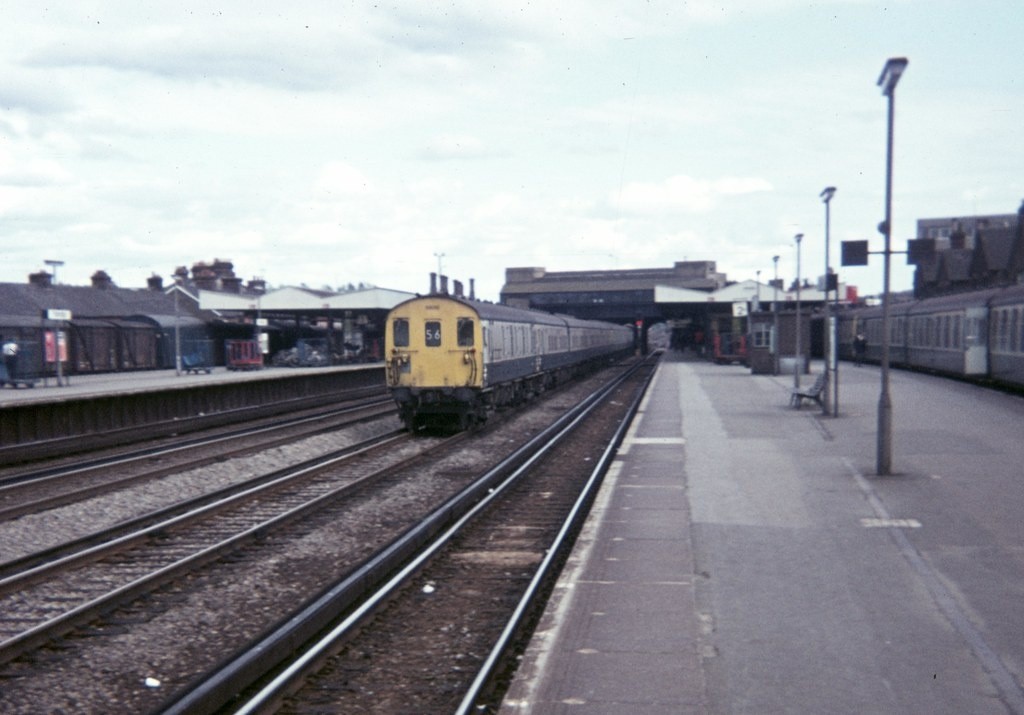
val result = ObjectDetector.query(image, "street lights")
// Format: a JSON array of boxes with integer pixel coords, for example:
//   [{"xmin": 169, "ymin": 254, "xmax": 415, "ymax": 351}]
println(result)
[
  {"xmin": 171, "ymin": 275, "xmax": 183, "ymax": 375},
  {"xmin": 772, "ymin": 256, "xmax": 781, "ymax": 310},
  {"xmin": 755, "ymin": 271, "xmax": 762, "ymax": 296},
  {"xmin": 794, "ymin": 234, "xmax": 806, "ymax": 387},
  {"xmin": 819, "ymin": 187, "xmax": 837, "ymax": 417},
  {"xmin": 44, "ymin": 260, "xmax": 65, "ymax": 386},
  {"xmin": 877, "ymin": 57, "xmax": 908, "ymax": 476}
]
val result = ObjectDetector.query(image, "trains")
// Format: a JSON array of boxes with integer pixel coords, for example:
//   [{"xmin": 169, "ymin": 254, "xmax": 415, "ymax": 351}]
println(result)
[
  {"xmin": 1, "ymin": 314, "xmax": 345, "ymax": 378},
  {"xmin": 385, "ymin": 272, "xmax": 641, "ymax": 436},
  {"xmin": 813, "ymin": 286, "xmax": 1024, "ymax": 396}
]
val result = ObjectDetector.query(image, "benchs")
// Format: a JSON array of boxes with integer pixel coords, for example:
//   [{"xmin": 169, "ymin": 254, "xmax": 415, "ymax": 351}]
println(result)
[
  {"xmin": 789, "ymin": 373, "xmax": 826, "ymax": 411},
  {"xmin": 181, "ymin": 353, "xmax": 212, "ymax": 375}
]
